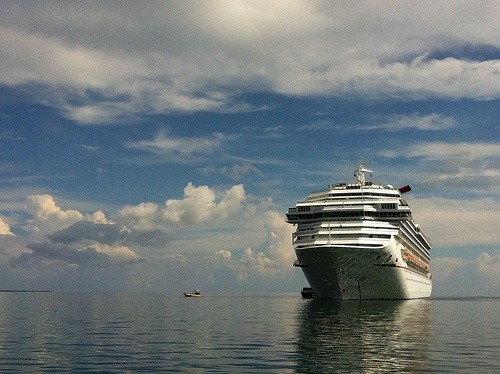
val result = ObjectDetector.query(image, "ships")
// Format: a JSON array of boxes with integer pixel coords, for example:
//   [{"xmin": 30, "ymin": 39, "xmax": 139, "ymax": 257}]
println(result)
[{"xmin": 284, "ymin": 156, "xmax": 433, "ymax": 301}]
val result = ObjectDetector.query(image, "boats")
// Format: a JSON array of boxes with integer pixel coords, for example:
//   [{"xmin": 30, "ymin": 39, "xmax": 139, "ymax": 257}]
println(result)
[
  {"xmin": 300, "ymin": 287, "xmax": 316, "ymax": 298},
  {"xmin": 183, "ymin": 289, "xmax": 205, "ymax": 297}
]
